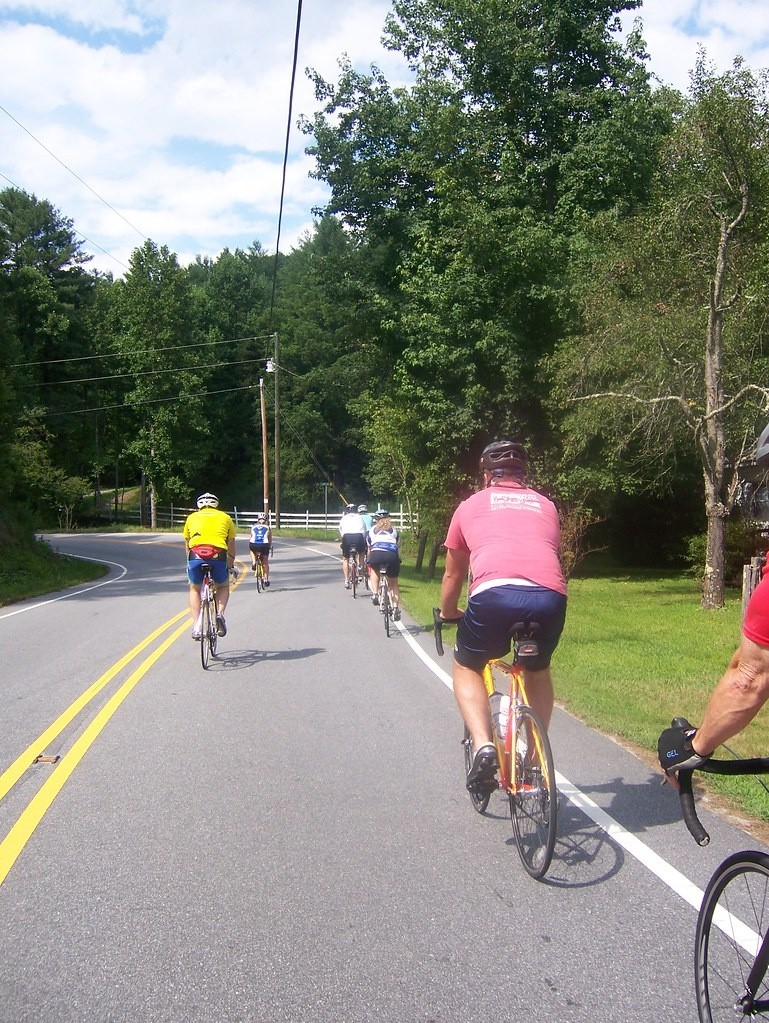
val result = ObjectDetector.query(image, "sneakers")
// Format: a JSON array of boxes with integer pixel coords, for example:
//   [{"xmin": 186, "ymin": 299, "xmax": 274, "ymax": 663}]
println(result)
[{"xmin": 466, "ymin": 742, "xmax": 500, "ymax": 793}]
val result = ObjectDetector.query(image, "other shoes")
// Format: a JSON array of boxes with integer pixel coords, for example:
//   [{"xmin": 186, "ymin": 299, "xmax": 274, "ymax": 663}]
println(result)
[
  {"xmin": 265, "ymin": 581, "xmax": 270, "ymax": 585},
  {"xmin": 392, "ymin": 607, "xmax": 401, "ymax": 621},
  {"xmin": 216, "ymin": 615, "xmax": 226, "ymax": 637},
  {"xmin": 192, "ymin": 628, "xmax": 205, "ymax": 638},
  {"xmin": 252, "ymin": 563, "xmax": 256, "ymax": 570},
  {"xmin": 518, "ymin": 765, "xmax": 545, "ymax": 792},
  {"xmin": 371, "ymin": 592, "xmax": 379, "ymax": 605},
  {"xmin": 344, "ymin": 582, "xmax": 350, "ymax": 588}
]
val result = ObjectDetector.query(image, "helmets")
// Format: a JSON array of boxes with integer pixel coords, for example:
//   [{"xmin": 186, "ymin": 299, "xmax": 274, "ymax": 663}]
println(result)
[
  {"xmin": 479, "ymin": 440, "xmax": 529, "ymax": 475},
  {"xmin": 196, "ymin": 492, "xmax": 218, "ymax": 509},
  {"xmin": 357, "ymin": 505, "xmax": 368, "ymax": 512},
  {"xmin": 375, "ymin": 509, "xmax": 390, "ymax": 520},
  {"xmin": 346, "ymin": 503, "xmax": 357, "ymax": 512},
  {"xmin": 258, "ymin": 513, "xmax": 267, "ymax": 521}
]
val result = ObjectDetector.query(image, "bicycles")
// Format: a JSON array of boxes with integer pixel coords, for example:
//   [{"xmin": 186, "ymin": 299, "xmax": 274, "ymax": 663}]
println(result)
[
  {"xmin": 660, "ymin": 717, "xmax": 769, "ymax": 1023},
  {"xmin": 431, "ymin": 607, "xmax": 558, "ymax": 880},
  {"xmin": 193, "ymin": 563, "xmax": 239, "ymax": 670},
  {"xmin": 359, "ymin": 547, "xmax": 370, "ymax": 590},
  {"xmin": 346, "ymin": 544, "xmax": 363, "ymax": 599},
  {"xmin": 374, "ymin": 564, "xmax": 400, "ymax": 637},
  {"xmin": 252, "ymin": 546, "xmax": 273, "ymax": 593}
]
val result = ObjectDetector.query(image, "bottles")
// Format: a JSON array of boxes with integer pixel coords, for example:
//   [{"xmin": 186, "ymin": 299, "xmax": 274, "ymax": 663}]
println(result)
[
  {"xmin": 489, "ymin": 690, "xmax": 504, "ymax": 739},
  {"xmin": 499, "ymin": 695, "xmax": 510, "ymax": 739}
]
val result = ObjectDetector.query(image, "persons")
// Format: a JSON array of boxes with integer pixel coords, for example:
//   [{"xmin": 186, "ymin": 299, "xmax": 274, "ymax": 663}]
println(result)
[
  {"xmin": 658, "ymin": 550, "xmax": 769, "ymax": 789},
  {"xmin": 183, "ymin": 492, "xmax": 237, "ymax": 638},
  {"xmin": 366, "ymin": 509, "xmax": 402, "ymax": 621},
  {"xmin": 440, "ymin": 441, "xmax": 567, "ymax": 794},
  {"xmin": 248, "ymin": 512, "xmax": 273, "ymax": 587},
  {"xmin": 339, "ymin": 504, "xmax": 374, "ymax": 589}
]
